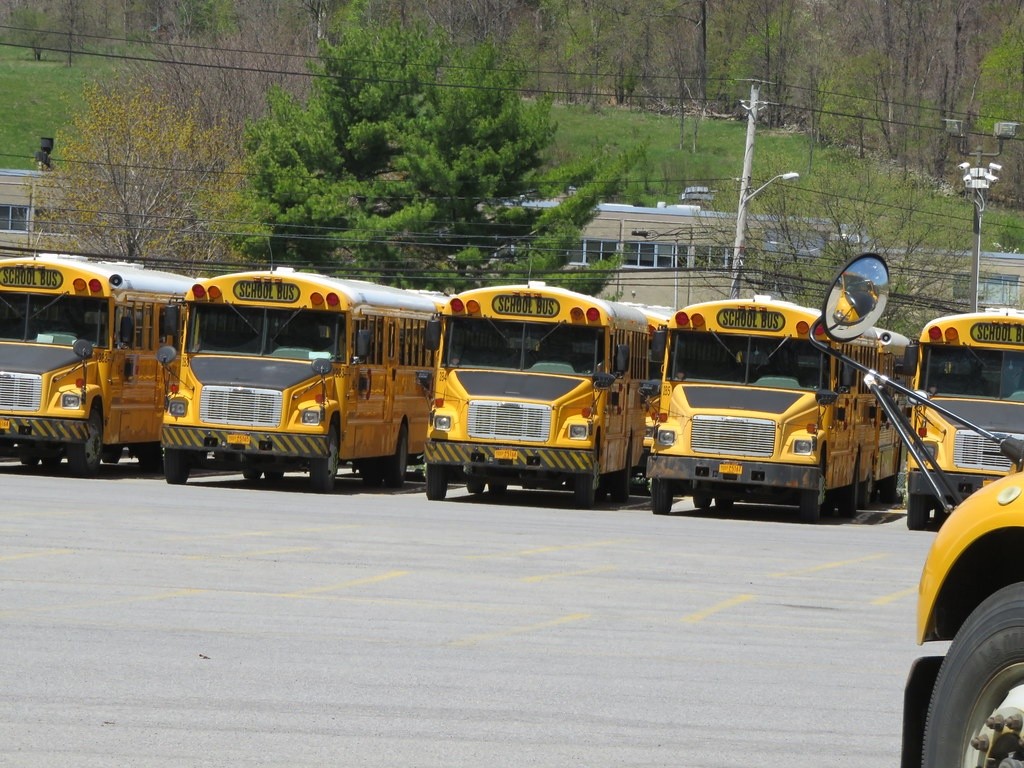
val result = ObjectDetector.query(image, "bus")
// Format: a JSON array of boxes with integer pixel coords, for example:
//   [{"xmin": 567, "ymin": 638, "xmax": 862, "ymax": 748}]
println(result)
[
  {"xmin": 1, "ymin": 252, "xmax": 214, "ymax": 479},
  {"xmin": 808, "ymin": 250, "xmax": 1023, "ymax": 768},
  {"xmin": 413, "ymin": 278, "xmax": 678, "ymax": 509},
  {"xmin": 902, "ymin": 310, "xmax": 1024, "ymax": 533},
  {"xmin": 156, "ymin": 264, "xmax": 458, "ymax": 494},
  {"xmin": 634, "ymin": 292, "xmax": 916, "ymax": 524}
]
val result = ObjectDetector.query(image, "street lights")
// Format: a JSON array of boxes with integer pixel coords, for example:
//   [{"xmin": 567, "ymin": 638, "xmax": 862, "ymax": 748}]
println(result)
[
  {"xmin": 728, "ymin": 171, "xmax": 799, "ymax": 301},
  {"xmin": 942, "ymin": 118, "xmax": 1020, "ymax": 313}
]
